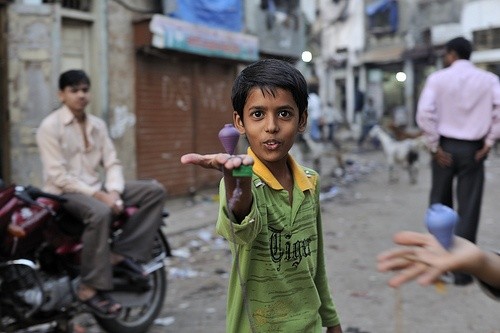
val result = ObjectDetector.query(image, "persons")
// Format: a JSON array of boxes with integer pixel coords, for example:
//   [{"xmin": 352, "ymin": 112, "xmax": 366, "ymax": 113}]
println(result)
[
  {"xmin": 357, "ymin": 98, "xmax": 408, "ymax": 149},
  {"xmin": 181, "ymin": 60, "xmax": 347, "ymax": 333},
  {"xmin": 375, "ymin": 231, "xmax": 500, "ymax": 300},
  {"xmin": 415, "ymin": 37, "xmax": 500, "ymax": 285},
  {"xmin": 36, "ymin": 70, "xmax": 166, "ymax": 319}
]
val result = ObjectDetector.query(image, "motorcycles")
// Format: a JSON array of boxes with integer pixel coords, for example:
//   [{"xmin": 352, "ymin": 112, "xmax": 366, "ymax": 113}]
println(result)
[{"xmin": 0, "ymin": 183, "xmax": 172, "ymax": 333}]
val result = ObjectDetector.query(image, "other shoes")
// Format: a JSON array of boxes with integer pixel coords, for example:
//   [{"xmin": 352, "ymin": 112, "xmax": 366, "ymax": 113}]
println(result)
[{"xmin": 454, "ymin": 278, "xmax": 474, "ymax": 286}]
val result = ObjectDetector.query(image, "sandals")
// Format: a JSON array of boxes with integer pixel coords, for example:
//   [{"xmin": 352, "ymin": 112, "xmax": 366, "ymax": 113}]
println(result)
[
  {"xmin": 74, "ymin": 290, "xmax": 123, "ymax": 321},
  {"xmin": 106, "ymin": 256, "xmax": 148, "ymax": 278}
]
